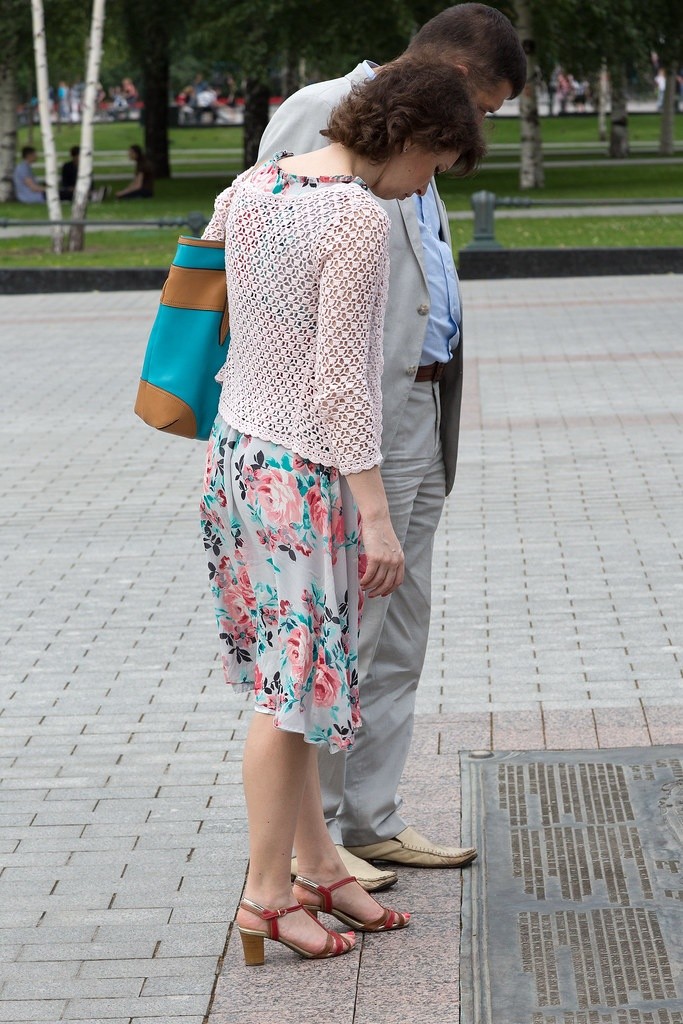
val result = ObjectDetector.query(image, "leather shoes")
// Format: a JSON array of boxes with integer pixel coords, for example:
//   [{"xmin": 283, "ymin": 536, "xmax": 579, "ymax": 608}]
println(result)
[
  {"xmin": 347, "ymin": 826, "xmax": 479, "ymax": 869},
  {"xmin": 291, "ymin": 845, "xmax": 397, "ymax": 892}
]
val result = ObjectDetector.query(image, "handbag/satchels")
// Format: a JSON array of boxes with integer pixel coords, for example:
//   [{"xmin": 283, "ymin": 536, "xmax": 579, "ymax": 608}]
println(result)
[{"xmin": 134, "ymin": 233, "xmax": 232, "ymax": 439}]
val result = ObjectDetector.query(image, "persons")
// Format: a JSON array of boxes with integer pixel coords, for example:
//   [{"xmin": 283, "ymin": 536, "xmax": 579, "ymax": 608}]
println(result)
[
  {"xmin": 12, "ymin": 146, "xmax": 113, "ymax": 204},
  {"xmin": 258, "ymin": 3, "xmax": 531, "ymax": 896},
  {"xmin": 46, "ymin": 66, "xmax": 681, "ymax": 119},
  {"xmin": 58, "ymin": 145, "xmax": 92, "ymax": 201},
  {"xmin": 195, "ymin": 55, "xmax": 491, "ymax": 966},
  {"xmin": 109, "ymin": 145, "xmax": 155, "ymax": 199}
]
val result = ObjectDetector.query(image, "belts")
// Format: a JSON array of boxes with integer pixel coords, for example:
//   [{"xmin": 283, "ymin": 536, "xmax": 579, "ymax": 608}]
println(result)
[{"xmin": 415, "ymin": 363, "xmax": 447, "ymax": 382}]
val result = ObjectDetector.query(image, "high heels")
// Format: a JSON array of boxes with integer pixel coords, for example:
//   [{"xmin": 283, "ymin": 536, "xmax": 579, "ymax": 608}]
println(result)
[
  {"xmin": 237, "ymin": 896, "xmax": 357, "ymax": 965},
  {"xmin": 293, "ymin": 874, "xmax": 412, "ymax": 931}
]
